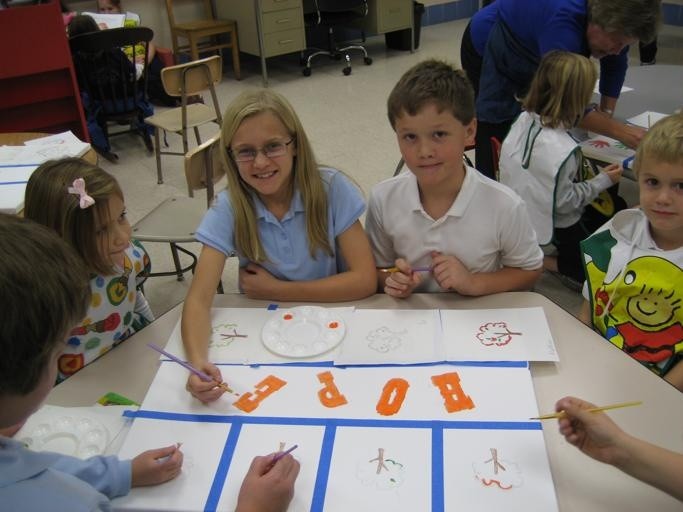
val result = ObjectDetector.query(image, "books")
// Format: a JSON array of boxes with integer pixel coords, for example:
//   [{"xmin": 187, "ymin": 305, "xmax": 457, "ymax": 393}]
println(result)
[{"xmin": 0, "ymin": 131, "xmax": 91, "ymax": 214}]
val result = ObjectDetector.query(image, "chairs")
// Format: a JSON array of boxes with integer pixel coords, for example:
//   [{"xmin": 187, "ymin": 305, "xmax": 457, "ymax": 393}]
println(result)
[
  {"xmin": 165, "ymin": 0, "xmax": 241, "ymax": 80},
  {"xmin": 143, "ymin": 55, "xmax": 223, "ymax": 198},
  {"xmin": 129, "ymin": 132, "xmax": 224, "ymax": 294},
  {"xmin": 303, "ymin": 1, "xmax": 372, "ymax": 77},
  {"xmin": 392, "ymin": 139, "xmax": 476, "ymax": 177},
  {"xmin": 490, "ymin": 137, "xmax": 502, "ymax": 183}
]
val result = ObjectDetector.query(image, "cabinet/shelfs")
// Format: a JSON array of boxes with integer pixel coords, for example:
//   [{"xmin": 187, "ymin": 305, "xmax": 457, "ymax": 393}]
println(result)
[{"xmin": 1, "ymin": 2, "xmax": 92, "ymax": 147}]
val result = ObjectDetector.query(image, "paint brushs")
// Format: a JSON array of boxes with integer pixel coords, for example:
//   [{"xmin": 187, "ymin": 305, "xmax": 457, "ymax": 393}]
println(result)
[
  {"xmin": 645, "ymin": 112, "xmax": 656, "ymax": 133},
  {"xmin": 155, "ymin": 443, "xmax": 190, "ymax": 468},
  {"xmin": 275, "ymin": 441, "xmax": 298, "ymax": 464},
  {"xmin": 143, "ymin": 341, "xmax": 238, "ymax": 396},
  {"xmin": 534, "ymin": 397, "xmax": 642, "ymax": 422},
  {"xmin": 377, "ymin": 266, "xmax": 438, "ymax": 274}
]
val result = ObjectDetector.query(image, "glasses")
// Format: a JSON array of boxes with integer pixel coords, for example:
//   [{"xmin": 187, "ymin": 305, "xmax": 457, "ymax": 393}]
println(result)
[{"xmin": 231, "ymin": 137, "xmax": 294, "ymax": 162}]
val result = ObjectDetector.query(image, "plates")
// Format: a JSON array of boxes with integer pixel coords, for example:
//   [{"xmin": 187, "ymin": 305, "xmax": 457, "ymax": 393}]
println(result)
[
  {"xmin": 258, "ymin": 304, "xmax": 346, "ymax": 359},
  {"xmin": 15, "ymin": 412, "xmax": 110, "ymax": 462}
]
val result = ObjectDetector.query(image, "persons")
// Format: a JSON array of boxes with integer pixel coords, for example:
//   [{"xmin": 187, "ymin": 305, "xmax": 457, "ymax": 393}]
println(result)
[
  {"xmin": 0, "ymin": 212, "xmax": 301, "ymax": 512},
  {"xmin": 578, "ymin": 114, "xmax": 682, "ymax": 392},
  {"xmin": 364, "ymin": 59, "xmax": 544, "ymax": 297},
  {"xmin": 639, "ymin": 37, "xmax": 657, "ymax": 66},
  {"xmin": 500, "ymin": 50, "xmax": 623, "ymax": 276},
  {"xmin": 23, "ymin": 158, "xmax": 155, "ymax": 386},
  {"xmin": 96, "ymin": 1, "xmax": 155, "ymax": 80},
  {"xmin": 459, "ymin": 0, "xmax": 665, "ymax": 184},
  {"xmin": 556, "ymin": 396, "xmax": 682, "ymax": 503},
  {"xmin": 180, "ymin": 88, "xmax": 378, "ymax": 403},
  {"xmin": 28, "ymin": 445, "xmax": 183, "ymax": 499}
]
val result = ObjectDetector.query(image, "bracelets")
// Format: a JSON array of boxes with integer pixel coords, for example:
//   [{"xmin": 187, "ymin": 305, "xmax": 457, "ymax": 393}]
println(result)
[{"xmin": 599, "ymin": 106, "xmax": 616, "ymax": 117}]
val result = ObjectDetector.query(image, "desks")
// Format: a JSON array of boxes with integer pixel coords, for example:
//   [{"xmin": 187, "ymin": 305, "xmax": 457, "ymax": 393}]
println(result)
[
  {"xmin": 565, "ymin": 65, "xmax": 683, "ymax": 182},
  {"xmin": 215, "ymin": 1, "xmax": 416, "ymax": 90},
  {"xmin": 43, "ymin": 291, "xmax": 683, "ymax": 511},
  {"xmin": 0, "ymin": 133, "xmax": 99, "ymax": 219}
]
